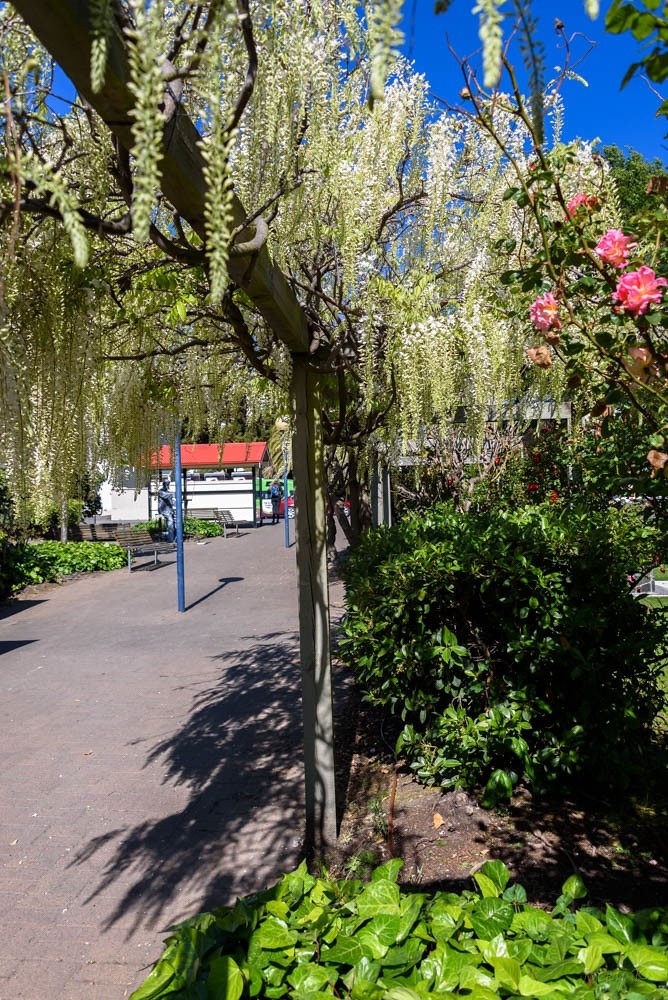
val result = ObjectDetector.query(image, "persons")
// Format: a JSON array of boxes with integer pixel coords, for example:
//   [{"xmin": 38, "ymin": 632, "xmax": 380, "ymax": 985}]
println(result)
[
  {"xmin": 270, "ymin": 479, "xmax": 282, "ymax": 522},
  {"xmin": 158, "ymin": 480, "xmax": 175, "ymax": 542}
]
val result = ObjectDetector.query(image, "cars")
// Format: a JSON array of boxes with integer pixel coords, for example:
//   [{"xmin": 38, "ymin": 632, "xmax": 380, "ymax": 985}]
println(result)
[{"xmin": 279, "ymin": 493, "xmax": 351, "ymax": 518}]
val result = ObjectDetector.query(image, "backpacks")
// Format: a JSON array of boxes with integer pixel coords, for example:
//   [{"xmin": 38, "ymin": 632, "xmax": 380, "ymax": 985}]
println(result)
[{"xmin": 272, "ymin": 485, "xmax": 278, "ymax": 494}]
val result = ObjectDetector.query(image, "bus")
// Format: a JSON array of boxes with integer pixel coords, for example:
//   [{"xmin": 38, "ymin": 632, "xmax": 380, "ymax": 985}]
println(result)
[{"xmin": 255, "ymin": 477, "xmax": 296, "ymax": 520}]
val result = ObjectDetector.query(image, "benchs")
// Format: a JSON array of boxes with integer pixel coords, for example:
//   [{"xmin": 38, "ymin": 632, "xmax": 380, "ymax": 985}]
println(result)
[
  {"xmin": 79, "ymin": 522, "xmax": 131, "ymax": 541},
  {"xmin": 173, "ymin": 507, "xmax": 223, "ymax": 526},
  {"xmin": 212, "ymin": 510, "xmax": 246, "ymax": 539},
  {"xmin": 112, "ymin": 529, "xmax": 176, "ymax": 574}
]
